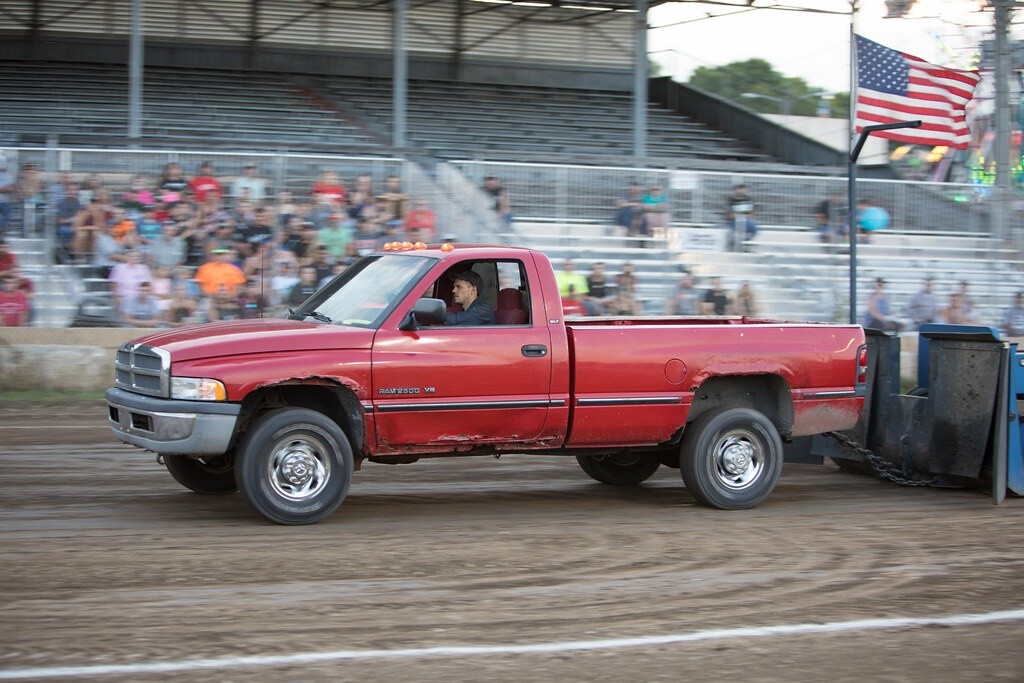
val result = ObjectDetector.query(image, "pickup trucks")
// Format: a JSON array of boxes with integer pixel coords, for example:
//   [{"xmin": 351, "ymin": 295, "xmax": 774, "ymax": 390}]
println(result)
[{"xmin": 106, "ymin": 243, "xmax": 869, "ymax": 524}]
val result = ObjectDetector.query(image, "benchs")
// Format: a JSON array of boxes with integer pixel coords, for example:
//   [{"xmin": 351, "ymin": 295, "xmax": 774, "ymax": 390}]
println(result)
[{"xmin": 0, "ymin": 61, "xmax": 1023, "ymax": 335}]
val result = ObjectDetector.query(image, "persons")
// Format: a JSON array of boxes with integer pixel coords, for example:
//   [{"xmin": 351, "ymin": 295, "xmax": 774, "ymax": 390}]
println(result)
[
  {"xmin": 477, "ymin": 175, "xmax": 512, "ymax": 229},
  {"xmin": 815, "ymin": 198, "xmax": 890, "ymax": 244},
  {"xmin": 725, "ymin": 184, "xmax": 756, "ymax": 244},
  {"xmin": 557, "ymin": 260, "xmax": 636, "ymax": 316},
  {"xmin": 673, "ymin": 270, "xmax": 757, "ymax": 315},
  {"xmin": 444, "ymin": 270, "xmax": 496, "ymax": 326},
  {"xmin": 906, "ymin": 274, "xmax": 994, "ymax": 329},
  {"xmin": 1001, "ymin": 292, "xmax": 1024, "ymax": 337},
  {"xmin": 616, "ymin": 178, "xmax": 670, "ymax": 238},
  {"xmin": 863, "ymin": 276, "xmax": 905, "ymax": 332},
  {"xmin": 0, "ymin": 159, "xmax": 434, "ymax": 325}
]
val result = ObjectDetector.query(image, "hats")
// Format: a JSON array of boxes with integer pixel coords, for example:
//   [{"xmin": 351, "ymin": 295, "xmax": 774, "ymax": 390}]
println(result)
[{"xmin": 448, "ymin": 269, "xmax": 483, "ymax": 295}]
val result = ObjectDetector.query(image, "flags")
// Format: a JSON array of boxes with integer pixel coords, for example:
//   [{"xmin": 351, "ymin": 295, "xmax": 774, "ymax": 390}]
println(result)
[{"xmin": 850, "ymin": 30, "xmax": 982, "ymax": 148}]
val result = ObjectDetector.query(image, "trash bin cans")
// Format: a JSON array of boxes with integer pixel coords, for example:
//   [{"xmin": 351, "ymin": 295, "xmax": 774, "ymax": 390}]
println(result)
[{"xmin": 23, "ymin": 195, "xmax": 45, "ymax": 238}]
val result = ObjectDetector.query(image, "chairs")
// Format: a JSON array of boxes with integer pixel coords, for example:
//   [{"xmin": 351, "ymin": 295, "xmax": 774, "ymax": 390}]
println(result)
[
  {"xmin": 446, "ymin": 296, "xmax": 463, "ymax": 313},
  {"xmin": 490, "ymin": 288, "xmax": 529, "ymax": 325}
]
